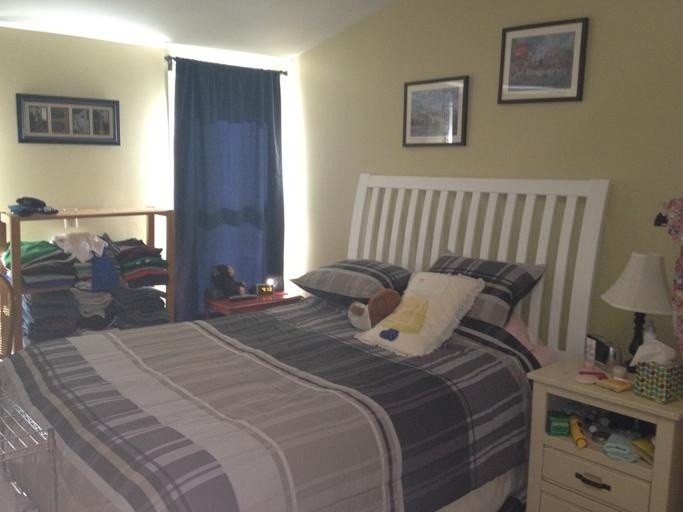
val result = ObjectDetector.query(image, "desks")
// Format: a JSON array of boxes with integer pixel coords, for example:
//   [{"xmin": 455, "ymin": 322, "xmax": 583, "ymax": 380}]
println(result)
[{"xmin": 204, "ymin": 290, "xmax": 302, "ymax": 318}]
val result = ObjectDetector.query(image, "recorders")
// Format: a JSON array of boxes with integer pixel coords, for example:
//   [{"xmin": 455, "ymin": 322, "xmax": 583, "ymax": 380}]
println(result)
[{"xmin": 585, "ymin": 334, "xmax": 622, "ymax": 373}]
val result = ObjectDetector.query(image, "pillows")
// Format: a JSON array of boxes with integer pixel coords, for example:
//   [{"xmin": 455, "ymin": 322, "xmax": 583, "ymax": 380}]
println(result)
[
  {"xmin": 427, "ymin": 246, "xmax": 548, "ymax": 331},
  {"xmin": 289, "ymin": 259, "xmax": 411, "ymax": 308},
  {"xmin": 351, "ymin": 270, "xmax": 485, "ymax": 357}
]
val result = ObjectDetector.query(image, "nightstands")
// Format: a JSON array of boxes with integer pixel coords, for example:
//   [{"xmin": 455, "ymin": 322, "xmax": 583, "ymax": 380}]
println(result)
[{"xmin": 523, "ymin": 354, "xmax": 682, "ymax": 511}]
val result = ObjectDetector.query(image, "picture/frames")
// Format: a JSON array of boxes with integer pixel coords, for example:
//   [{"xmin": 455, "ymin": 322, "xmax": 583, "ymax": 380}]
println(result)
[
  {"xmin": 495, "ymin": 16, "xmax": 589, "ymax": 104},
  {"xmin": 14, "ymin": 92, "xmax": 120, "ymax": 146},
  {"xmin": 401, "ymin": 75, "xmax": 471, "ymax": 148}
]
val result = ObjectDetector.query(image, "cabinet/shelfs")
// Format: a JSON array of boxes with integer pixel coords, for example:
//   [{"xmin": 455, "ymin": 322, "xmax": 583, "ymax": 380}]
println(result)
[{"xmin": 0, "ymin": 205, "xmax": 176, "ymax": 351}]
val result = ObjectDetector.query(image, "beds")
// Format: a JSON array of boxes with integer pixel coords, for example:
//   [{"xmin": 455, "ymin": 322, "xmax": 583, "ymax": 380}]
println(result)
[{"xmin": 0, "ymin": 170, "xmax": 610, "ymax": 512}]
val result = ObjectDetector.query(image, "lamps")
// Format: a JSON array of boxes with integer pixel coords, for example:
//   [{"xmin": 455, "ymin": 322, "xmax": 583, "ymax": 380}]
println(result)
[{"xmin": 600, "ymin": 251, "xmax": 676, "ymax": 372}]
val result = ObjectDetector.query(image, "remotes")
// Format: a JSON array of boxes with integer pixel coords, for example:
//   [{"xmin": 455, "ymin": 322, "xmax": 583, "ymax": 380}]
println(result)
[{"xmin": 229, "ymin": 294, "xmax": 257, "ymax": 301}]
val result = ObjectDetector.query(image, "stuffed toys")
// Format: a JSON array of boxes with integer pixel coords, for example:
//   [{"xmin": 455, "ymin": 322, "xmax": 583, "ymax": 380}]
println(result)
[
  {"xmin": 212, "ymin": 264, "xmax": 247, "ymax": 296},
  {"xmin": 348, "ymin": 288, "xmax": 401, "ymax": 330}
]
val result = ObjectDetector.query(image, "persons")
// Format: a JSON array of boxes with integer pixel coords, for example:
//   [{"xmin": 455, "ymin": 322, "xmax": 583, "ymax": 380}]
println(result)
[{"xmin": 26, "ymin": 104, "xmax": 109, "ymax": 134}]
